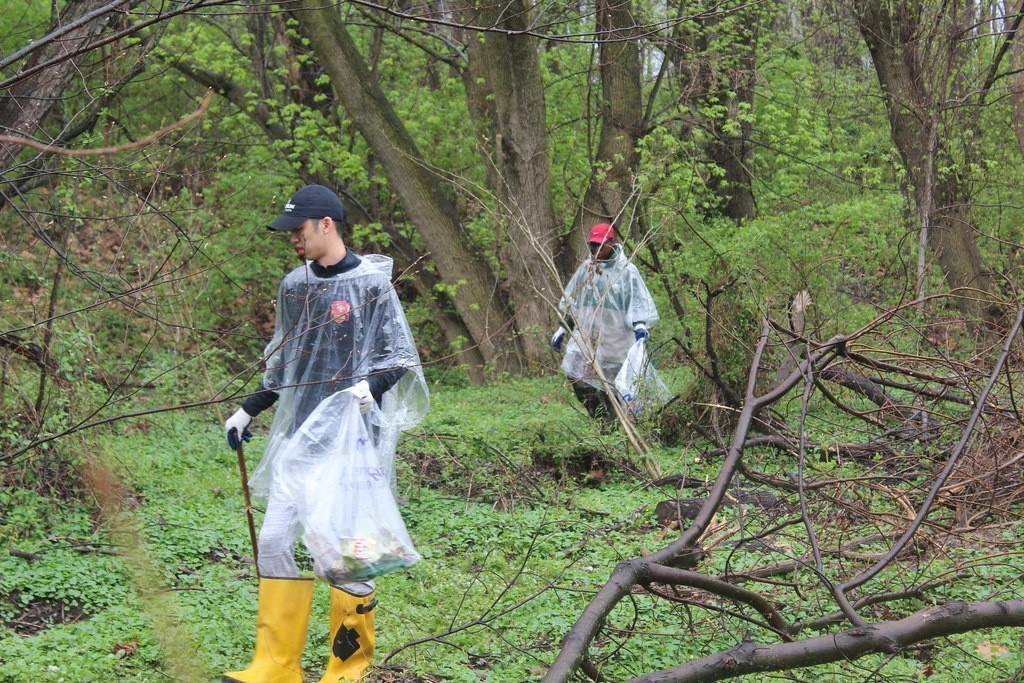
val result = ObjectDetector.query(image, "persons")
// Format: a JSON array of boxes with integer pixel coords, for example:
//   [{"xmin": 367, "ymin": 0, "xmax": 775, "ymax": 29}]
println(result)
[
  {"xmin": 550, "ymin": 223, "xmax": 650, "ymax": 435},
  {"xmin": 220, "ymin": 184, "xmax": 412, "ymax": 683}
]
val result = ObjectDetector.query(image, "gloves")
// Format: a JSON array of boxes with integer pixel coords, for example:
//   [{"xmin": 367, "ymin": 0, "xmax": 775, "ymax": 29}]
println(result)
[
  {"xmin": 344, "ymin": 380, "xmax": 373, "ymax": 414},
  {"xmin": 633, "ymin": 324, "xmax": 650, "ymax": 343},
  {"xmin": 551, "ymin": 326, "xmax": 566, "ymax": 353},
  {"xmin": 223, "ymin": 406, "xmax": 252, "ymax": 450}
]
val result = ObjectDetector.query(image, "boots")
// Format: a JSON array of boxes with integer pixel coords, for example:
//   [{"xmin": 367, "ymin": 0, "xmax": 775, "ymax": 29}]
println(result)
[
  {"xmin": 222, "ymin": 576, "xmax": 314, "ymax": 683},
  {"xmin": 314, "ymin": 585, "xmax": 378, "ymax": 683}
]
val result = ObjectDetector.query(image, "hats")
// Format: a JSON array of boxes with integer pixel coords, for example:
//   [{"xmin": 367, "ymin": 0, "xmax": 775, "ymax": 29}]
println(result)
[
  {"xmin": 586, "ymin": 222, "xmax": 614, "ymax": 245},
  {"xmin": 265, "ymin": 184, "xmax": 346, "ymax": 231}
]
